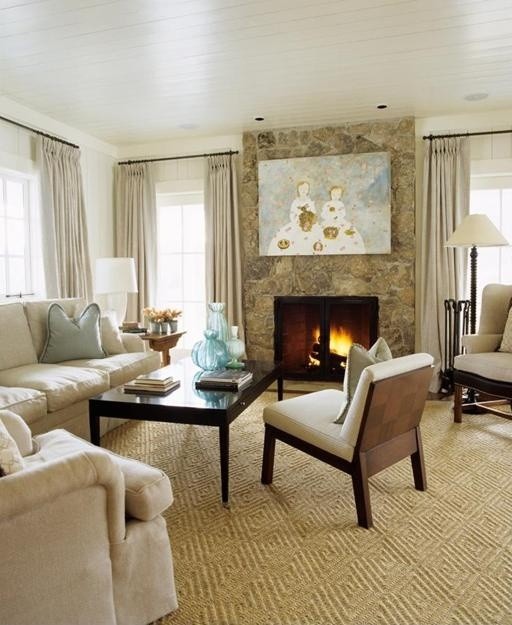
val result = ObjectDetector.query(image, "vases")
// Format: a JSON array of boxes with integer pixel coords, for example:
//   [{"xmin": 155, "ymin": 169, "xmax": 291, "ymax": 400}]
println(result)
[
  {"xmin": 207, "ymin": 302, "xmax": 226, "ymax": 340},
  {"xmin": 161, "ymin": 322, "xmax": 168, "ymax": 333},
  {"xmin": 170, "ymin": 321, "xmax": 178, "ymax": 332},
  {"xmin": 149, "ymin": 321, "xmax": 160, "ymax": 334},
  {"xmin": 226, "ymin": 325, "xmax": 247, "ymax": 370},
  {"xmin": 192, "ymin": 330, "xmax": 227, "ymax": 371}
]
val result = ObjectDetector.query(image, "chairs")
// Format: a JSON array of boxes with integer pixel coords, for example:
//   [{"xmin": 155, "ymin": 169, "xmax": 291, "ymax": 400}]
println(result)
[
  {"xmin": 0, "ymin": 409, "xmax": 180, "ymax": 624},
  {"xmin": 450, "ymin": 284, "xmax": 511, "ymax": 424},
  {"xmin": 260, "ymin": 351, "xmax": 434, "ymax": 529}
]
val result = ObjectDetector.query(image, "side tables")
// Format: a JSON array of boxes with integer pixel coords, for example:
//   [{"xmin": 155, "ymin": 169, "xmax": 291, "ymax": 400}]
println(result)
[{"xmin": 141, "ymin": 331, "xmax": 187, "ymax": 367}]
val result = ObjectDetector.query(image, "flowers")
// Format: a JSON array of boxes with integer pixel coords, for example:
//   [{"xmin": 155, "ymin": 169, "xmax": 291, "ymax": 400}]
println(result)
[{"xmin": 143, "ymin": 307, "xmax": 182, "ymax": 322}]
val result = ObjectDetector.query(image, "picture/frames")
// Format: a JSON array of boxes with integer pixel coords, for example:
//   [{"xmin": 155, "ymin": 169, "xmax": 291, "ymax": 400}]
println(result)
[{"xmin": 258, "ymin": 151, "xmax": 392, "ymax": 256}]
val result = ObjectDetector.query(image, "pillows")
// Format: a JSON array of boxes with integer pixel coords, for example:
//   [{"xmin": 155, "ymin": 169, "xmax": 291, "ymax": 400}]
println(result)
[
  {"xmin": 39, "ymin": 302, "xmax": 108, "ymax": 362},
  {"xmin": 499, "ymin": 308, "xmax": 512, "ymax": 352},
  {"xmin": 332, "ymin": 338, "xmax": 392, "ymax": 424},
  {"xmin": 1, "ymin": 409, "xmax": 35, "ymax": 458},
  {"xmin": 100, "ymin": 316, "xmax": 127, "ymax": 354}
]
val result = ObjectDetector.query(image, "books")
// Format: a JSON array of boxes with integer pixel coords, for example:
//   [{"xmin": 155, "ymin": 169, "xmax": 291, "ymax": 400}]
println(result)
[
  {"xmin": 123, "ymin": 380, "xmax": 180, "ymax": 392},
  {"xmin": 134, "ymin": 374, "xmax": 173, "ymax": 386},
  {"xmin": 119, "ymin": 322, "xmax": 149, "ymax": 336},
  {"xmin": 199, "ymin": 371, "xmax": 253, "ymax": 383},
  {"xmin": 195, "ymin": 377, "xmax": 254, "ymax": 392},
  {"xmin": 124, "ymin": 383, "xmax": 180, "ymax": 396}
]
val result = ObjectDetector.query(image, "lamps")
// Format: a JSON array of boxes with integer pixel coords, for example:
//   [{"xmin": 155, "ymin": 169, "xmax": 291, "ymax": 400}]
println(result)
[
  {"xmin": 442, "ymin": 213, "xmax": 509, "ymax": 416},
  {"xmin": 95, "ymin": 257, "xmax": 139, "ymax": 327}
]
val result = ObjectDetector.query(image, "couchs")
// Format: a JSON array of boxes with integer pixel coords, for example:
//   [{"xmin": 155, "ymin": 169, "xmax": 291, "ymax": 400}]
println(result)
[{"xmin": 0, "ymin": 298, "xmax": 163, "ymax": 441}]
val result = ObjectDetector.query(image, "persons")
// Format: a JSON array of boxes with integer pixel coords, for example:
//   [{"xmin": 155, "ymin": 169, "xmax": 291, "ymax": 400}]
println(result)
[
  {"xmin": 319, "ymin": 186, "xmax": 365, "ymax": 254},
  {"xmin": 267, "ymin": 181, "xmax": 320, "ymax": 255}
]
